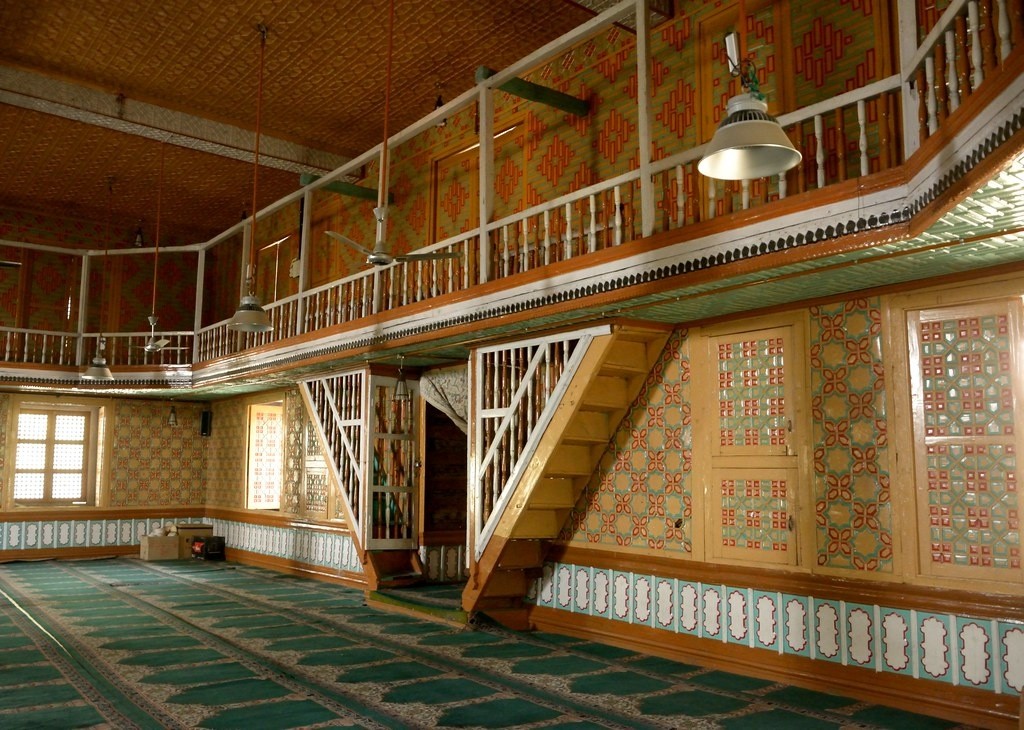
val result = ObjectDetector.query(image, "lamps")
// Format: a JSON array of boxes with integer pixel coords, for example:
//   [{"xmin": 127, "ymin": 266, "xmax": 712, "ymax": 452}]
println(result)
[
  {"xmin": 80, "ymin": 184, "xmax": 115, "ymax": 381},
  {"xmin": 166, "ymin": 397, "xmax": 178, "ymax": 427},
  {"xmin": 430, "ymin": 80, "xmax": 447, "ymax": 128},
  {"xmin": 697, "ymin": 0, "xmax": 804, "ymax": 182},
  {"xmin": 133, "ymin": 225, "xmax": 144, "ymax": 248},
  {"xmin": 226, "ymin": 22, "xmax": 275, "ymax": 333}
]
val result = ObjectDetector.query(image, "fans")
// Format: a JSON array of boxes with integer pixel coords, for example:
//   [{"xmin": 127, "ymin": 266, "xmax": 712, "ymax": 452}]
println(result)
[
  {"xmin": 322, "ymin": 0, "xmax": 463, "ymax": 267},
  {"xmin": 122, "ymin": 140, "xmax": 191, "ymax": 351}
]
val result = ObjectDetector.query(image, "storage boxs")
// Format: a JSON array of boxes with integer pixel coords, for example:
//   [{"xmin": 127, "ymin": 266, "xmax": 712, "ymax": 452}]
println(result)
[{"xmin": 139, "ymin": 525, "xmax": 226, "ymax": 562}]
